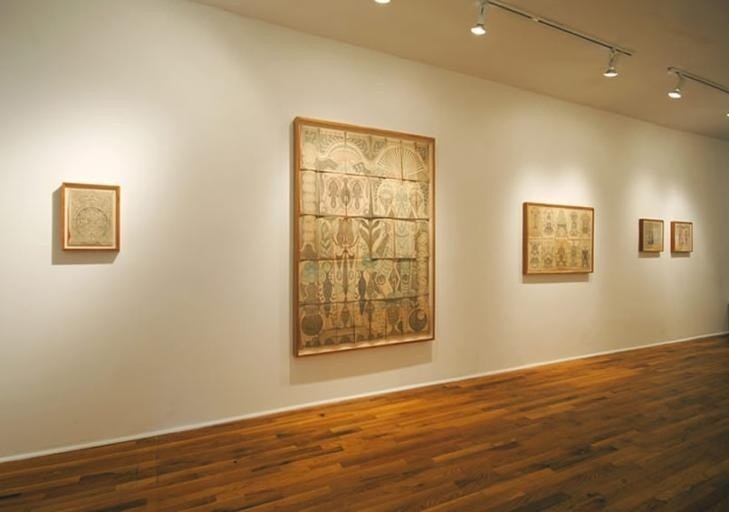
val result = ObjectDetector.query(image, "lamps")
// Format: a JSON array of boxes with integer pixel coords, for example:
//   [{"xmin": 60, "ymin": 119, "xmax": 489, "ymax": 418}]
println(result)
[
  {"xmin": 470, "ymin": 0, "xmax": 621, "ymax": 78},
  {"xmin": 668, "ymin": 71, "xmax": 684, "ymax": 100}
]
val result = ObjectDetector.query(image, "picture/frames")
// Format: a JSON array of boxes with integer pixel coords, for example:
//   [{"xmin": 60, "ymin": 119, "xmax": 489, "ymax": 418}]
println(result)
[
  {"xmin": 670, "ymin": 221, "xmax": 694, "ymax": 253},
  {"xmin": 291, "ymin": 117, "xmax": 435, "ymax": 359},
  {"xmin": 639, "ymin": 219, "xmax": 665, "ymax": 254},
  {"xmin": 522, "ymin": 201, "xmax": 594, "ymax": 276},
  {"xmin": 61, "ymin": 182, "xmax": 119, "ymax": 252}
]
